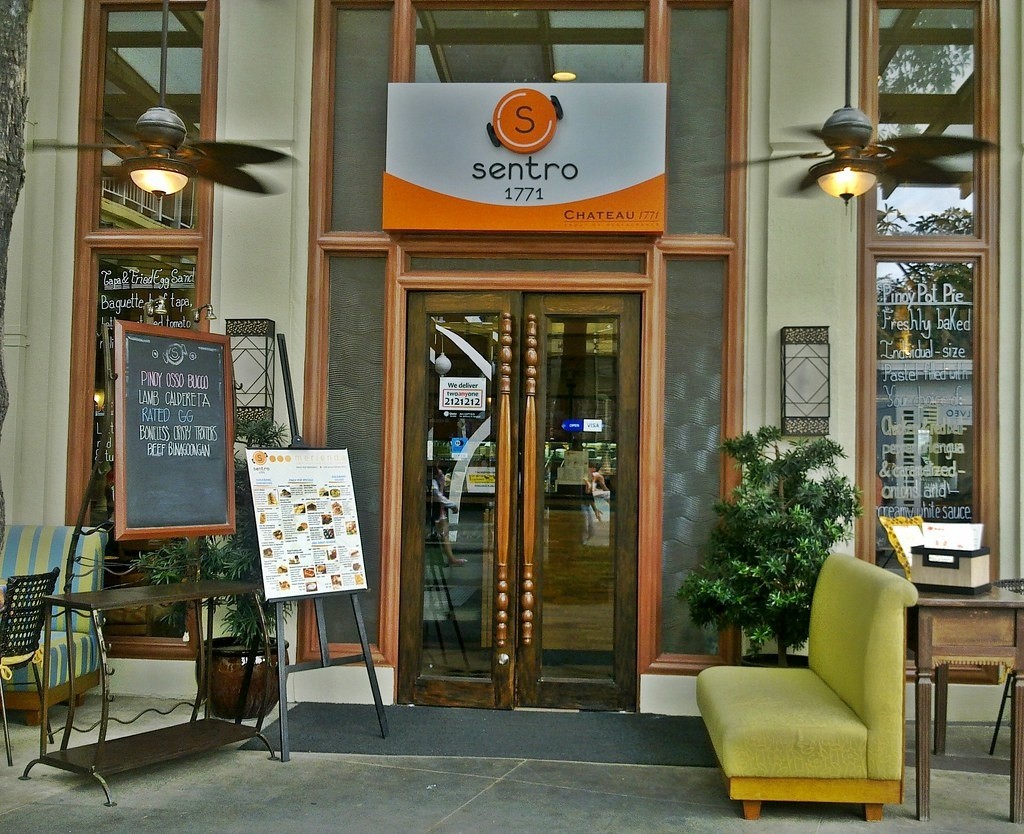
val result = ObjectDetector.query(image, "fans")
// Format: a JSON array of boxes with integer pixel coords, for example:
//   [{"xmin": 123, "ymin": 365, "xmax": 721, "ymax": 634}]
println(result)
[
  {"xmin": 700, "ymin": 0, "xmax": 1000, "ymax": 197},
  {"xmin": 27, "ymin": 1, "xmax": 300, "ymax": 196}
]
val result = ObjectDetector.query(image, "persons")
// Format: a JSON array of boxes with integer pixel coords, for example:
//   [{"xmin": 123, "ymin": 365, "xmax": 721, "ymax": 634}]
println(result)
[
  {"xmin": 583, "ymin": 460, "xmax": 610, "ymax": 539},
  {"xmin": 431, "ymin": 458, "xmax": 468, "ymax": 567}
]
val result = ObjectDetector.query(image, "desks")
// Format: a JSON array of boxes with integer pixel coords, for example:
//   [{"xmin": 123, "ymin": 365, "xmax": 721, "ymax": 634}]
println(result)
[{"xmin": 885, "ymin": 569, "xmax": 1024, "ymax": 823}]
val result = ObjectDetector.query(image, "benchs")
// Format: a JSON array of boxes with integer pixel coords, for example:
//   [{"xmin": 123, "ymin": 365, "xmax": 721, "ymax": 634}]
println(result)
[
  {"xmin": 696, "ymin": 554, "xmax": 919, "ymax": 821},
  {"xmin": 0, "ymin": 525, "xmax": 109, "ymax": 725}
]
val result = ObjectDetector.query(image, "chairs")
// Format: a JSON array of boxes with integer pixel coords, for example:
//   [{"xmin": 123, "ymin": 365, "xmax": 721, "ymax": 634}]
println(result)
[{"xmin": 0, "ymin": 567, "xmax": 60, "ymax": 767}]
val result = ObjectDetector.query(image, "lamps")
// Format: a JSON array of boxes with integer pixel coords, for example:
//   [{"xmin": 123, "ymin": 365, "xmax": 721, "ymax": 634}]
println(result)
[
  {"xmin": 225, "ymin": 318, "xmax": 275, "ymax": 425},
  {"xmin": 121, "ymin": 147, "xmax": 199, "ymax": 203},
  {"xmin": 779, "ymin": 326, "xmax": 829, "ymax": 436},
  {"xmin": 808, "ymin": 148, "xmax": 886, "ymax": 209},
  {"xmin": 435, "ymin": 332, "xmax": 451, "ymax": 377}
]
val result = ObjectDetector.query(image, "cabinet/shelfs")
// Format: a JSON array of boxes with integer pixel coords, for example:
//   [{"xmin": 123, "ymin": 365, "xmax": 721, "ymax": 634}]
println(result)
[{"xmin": 17, "ymin": 578, "xmax": 279, "ymax": 807}]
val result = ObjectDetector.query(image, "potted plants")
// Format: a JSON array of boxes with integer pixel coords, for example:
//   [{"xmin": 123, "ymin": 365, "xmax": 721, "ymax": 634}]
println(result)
[
  {"xmin": 130, "ymin": 418, "xmax": 292, "ymax": 720},
  {"xmin": 675, "ymin": 426, "xmax": 865, "ymax": 668}
]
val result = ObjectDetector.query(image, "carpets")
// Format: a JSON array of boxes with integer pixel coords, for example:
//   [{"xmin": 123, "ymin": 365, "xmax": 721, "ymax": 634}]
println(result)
[{"xmin": 238, "ymin": 702, "xmax": 719, "ymax": 768}]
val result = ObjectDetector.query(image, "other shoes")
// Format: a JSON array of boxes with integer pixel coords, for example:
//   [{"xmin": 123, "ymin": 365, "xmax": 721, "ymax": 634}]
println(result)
[{"xmin": 448, "ymin": 559, "xmax": 468, "ymax": 567}]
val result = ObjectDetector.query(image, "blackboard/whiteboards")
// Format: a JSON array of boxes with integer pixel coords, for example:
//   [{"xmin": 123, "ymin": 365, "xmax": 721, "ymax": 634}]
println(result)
[{"xmin": 112, "ymin": 318, "xmax": 238, "ymax": 540}]
